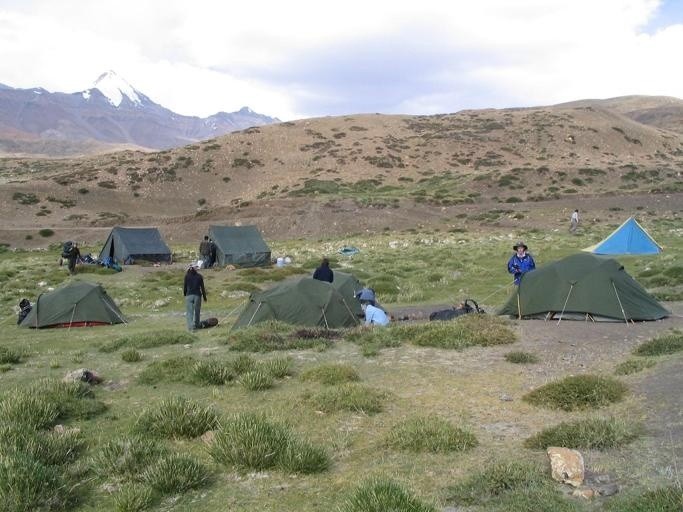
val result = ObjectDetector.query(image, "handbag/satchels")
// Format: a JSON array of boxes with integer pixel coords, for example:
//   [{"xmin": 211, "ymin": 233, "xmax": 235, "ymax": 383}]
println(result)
[
  {"xmin": 200, "ymin": 319, "xmax": 218, "ymax": 328},
  {"xmin": 428, "ymin": 307, "xmax": 468, "ymax": 322}
]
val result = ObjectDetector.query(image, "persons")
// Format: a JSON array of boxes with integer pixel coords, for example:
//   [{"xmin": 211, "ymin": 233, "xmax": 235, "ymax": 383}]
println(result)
[
  {"xmin": 360, "ymin": 302, "xmax": 388, "ymax": 326},
  {"xmin": 312, "ymin": 258, "xmax": 334, "ymax": 283},
  {"xmin": 66, "ymin": 242, "xmax": 83, "ymax": 273},
  {"xmin": 16, "ymin": 299, "xmax": 32, "ymax": 326},
  {"xmin": 507, "ymin": 240, "xmax": 535, "ymax": 286},
  {"xmin": 208, "ymin": 239, "xmax": 216, "ymax": 266},
  {"xmin": 568, "ymin": 208, "xmax": 578, "ymax": 232},
  {"xmin": 352, "ymin": 287, "xmax": 375, "ymax": 306},
  {"xmin": 182, "ymin": 263, "xmax": 208, "ymax": 332},
  {"xmin": 199, "ymin": 235, "xmax": 212, "ymax": 268}
]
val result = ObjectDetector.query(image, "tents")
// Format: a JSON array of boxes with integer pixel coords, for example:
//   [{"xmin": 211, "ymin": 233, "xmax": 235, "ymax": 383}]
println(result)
[
  {"xmin": 495, "ymin": 251, "xmax": 673, "ymax": 324},
  {"xmin": 579, "ymin": 216, "xmax": 663, "ymax": 256},
  {"xmin": 207, "ymin": 224, "xmax": 272, "ymax": 268},
  {"xmin": 97, "ymin": 225, "xmax": 170, "ymax": 265},
  {"xmin": 303, "ymin": 272, "xmax": 385, "ymax": 319},
  {"xmin": 17, "ymin": 280, "xmax": 127, "ymax": 329},
  {"xmin": 229, "ymin": 276, "xmax": 361, "ymax": 332}
]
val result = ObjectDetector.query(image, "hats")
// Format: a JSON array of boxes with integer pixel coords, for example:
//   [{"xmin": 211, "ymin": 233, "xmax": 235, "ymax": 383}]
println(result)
[{"xmin": 513, "ymin": 242, "xmax": 528, "ymax": 250}]
